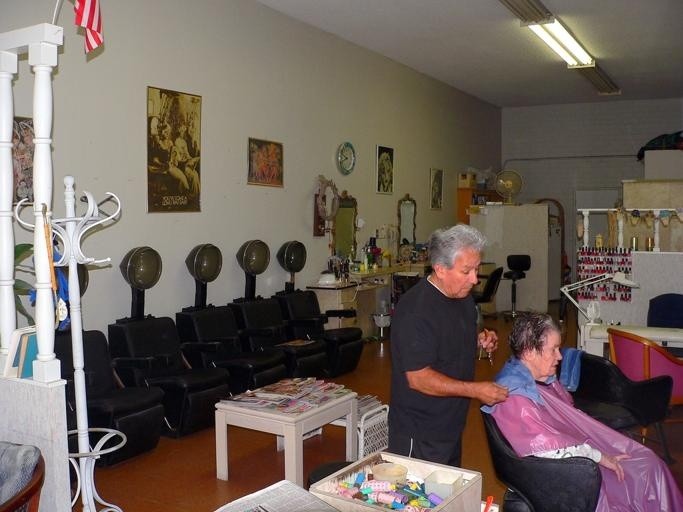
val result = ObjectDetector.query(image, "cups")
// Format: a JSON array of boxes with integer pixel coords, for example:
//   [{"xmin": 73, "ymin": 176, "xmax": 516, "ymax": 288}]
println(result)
[
  {"xmin": 646, "ymin": 237, "xmax": 654, "ymax": 250},
  {"xmin": 629, "ymin": 237, "xmax": 637, "ymax": 250}
]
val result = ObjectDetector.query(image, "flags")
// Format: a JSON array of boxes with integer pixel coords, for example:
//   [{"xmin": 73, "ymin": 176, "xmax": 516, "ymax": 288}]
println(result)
[{"xmin": 74, "ymin": 0, "xmax": 103, "ymax": 54}]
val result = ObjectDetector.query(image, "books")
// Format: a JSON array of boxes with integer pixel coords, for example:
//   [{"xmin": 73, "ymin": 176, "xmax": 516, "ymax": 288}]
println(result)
[
  {"xmin": 219, "ymin": 377, "xmax": 383, "ymax": 420},
  {"xmin": 3, "ymin": 325, "xmax": 38, "ymax": 380}
]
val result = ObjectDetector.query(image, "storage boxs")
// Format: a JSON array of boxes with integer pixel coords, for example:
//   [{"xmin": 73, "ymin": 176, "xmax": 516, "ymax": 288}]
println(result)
[
  {"xmin": 643, "ymin": 150, "xmax": 682, "ymax": 180},
  {"xmin": 308, "ymin": 451, "xmax": 481, "ymax": 512}
]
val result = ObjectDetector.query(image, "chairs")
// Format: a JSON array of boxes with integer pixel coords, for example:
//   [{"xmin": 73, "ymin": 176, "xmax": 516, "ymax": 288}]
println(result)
[
  {"xmin": 226, "ymin": 297, "xmax": 327, "ymax": 379},
  {"xmin": 472, "ymin": 266, "xmax": 503, "ymax": 333},
  {"xmin": 478, "ymin": 380, "xmax": 606, "ymax": 512},
  {"xmin": 0, "ymin": 439, "xmax": 46, "ymax": 512},
  {"xmin": 109, "ymin": 315, "xmax": 233, "ymax": 439},
  {"xmin": 502, "ymin": 253, "xmax": 532, "ymax": 320},
  {"xmin": 270, "ymin": 288, "xmax": 363, "ymax": 378},
  {"xmin": 606, "ymin": 327, "xmax": 682, "ymax": 449},
  {"xmin": 553, "ymin": 349, "xmax": 674, "ymax": 462},
  {"xmin": 176, "ymin": 304, "xmax": 288, "ymax": 390},
  {"xmin": 55, "ymin": 330, "xmax": 166, "ymax": 467},
  {"xmin": 644, "ymin": 291, "xmax": 683, "ymax": 360}
]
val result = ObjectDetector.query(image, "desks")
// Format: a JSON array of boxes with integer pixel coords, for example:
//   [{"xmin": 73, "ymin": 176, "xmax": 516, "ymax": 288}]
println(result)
[
  {"xmin": 348, "ymin": 264, "xmax": 409, "ymax": 342},
  {"xmin": 211, "ymin": 390, "xmax": 360, "ymax": 488},
  {"xmin": 589, "ymin": 324, "xmax": 682, "ymax": 361}
]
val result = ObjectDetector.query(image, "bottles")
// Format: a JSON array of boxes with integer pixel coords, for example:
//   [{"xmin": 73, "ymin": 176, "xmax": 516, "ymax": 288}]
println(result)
[
  {"xmin": 348, "ymin": 237, "xmax": 382, "ymax": 272},
  {"xmin": 412, "ymin": 247, "xmax": 427, "ymax": 263}
]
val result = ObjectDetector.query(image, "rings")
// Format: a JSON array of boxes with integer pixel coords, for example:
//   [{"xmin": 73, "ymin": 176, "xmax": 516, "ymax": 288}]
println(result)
[{"xmin": 491, "ymin": 338, "xmax": 497, "ymax": 345}]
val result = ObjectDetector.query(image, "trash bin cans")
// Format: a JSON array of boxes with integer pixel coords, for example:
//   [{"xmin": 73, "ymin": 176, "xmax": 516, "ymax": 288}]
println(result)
[{"xmin": 371, "ymin": 313, "xmax": 391, "ymax": 341}]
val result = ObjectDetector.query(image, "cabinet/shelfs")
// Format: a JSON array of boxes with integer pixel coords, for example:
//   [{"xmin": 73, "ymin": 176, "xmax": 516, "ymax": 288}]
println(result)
[
  {"xmin": 456, "ymin": 185, "xmax": 504, "ymax": 227},
  {"xmin": 402, "ymin": 262, "xmax": 431, "ymax": 279},
  {"xmin": 473, "ymin": 261, "xmax": 498, "ymax": 319},
  {"xmin": 304, "ymin": 280, "xmax": 363, "ymax": 342}
]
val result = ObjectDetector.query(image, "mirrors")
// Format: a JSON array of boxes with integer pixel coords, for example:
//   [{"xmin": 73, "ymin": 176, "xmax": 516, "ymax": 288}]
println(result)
[
  {"xmin": 330, "ymin": 190, "xmax": 357, "ymax": 259},
  {"xmin": 396, "ymin": 192, "xmax": 417, "ymax": 248}
]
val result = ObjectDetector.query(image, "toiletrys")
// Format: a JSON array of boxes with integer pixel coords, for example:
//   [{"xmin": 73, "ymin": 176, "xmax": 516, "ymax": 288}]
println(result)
[{"xmin": 577, "ymin": 247, "xmax": 632, "ymax": 302}]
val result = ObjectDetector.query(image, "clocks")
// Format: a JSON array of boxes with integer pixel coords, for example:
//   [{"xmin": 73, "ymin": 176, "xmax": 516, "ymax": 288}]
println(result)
[{"xmin": 336, "ymin": 142, "xmax": 355, "ymax": 175}]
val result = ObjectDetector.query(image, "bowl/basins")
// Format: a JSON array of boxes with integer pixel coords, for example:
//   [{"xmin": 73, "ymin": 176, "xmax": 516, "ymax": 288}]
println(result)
[{"xmin": 372, "ymin": 463, "xmax": 407, "ymax": 486}]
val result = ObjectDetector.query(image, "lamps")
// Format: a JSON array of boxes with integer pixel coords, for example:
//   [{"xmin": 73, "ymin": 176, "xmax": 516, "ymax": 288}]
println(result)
[
  {"xmin": 503, "ymin": 0, "xmax": 622, "ymax": 98},
  {"xmin": 559, "ymin": 271, "xmax": 640, "ymax": 325}
]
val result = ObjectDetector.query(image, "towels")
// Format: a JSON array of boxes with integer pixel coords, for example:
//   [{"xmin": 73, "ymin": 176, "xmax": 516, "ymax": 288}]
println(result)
[
  {"xmin": 559, "ymin": 347, "xmax": 584, "ymax": 391},
  {"xmin": 480, "ymin": 355, "xmax": 557, "ymax": 415}
]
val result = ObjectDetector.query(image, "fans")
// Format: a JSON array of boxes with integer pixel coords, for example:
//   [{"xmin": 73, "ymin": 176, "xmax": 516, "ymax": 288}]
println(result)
[{"xmin": 494, "ymin": 170, "xmax": 524, "ymax": 205}]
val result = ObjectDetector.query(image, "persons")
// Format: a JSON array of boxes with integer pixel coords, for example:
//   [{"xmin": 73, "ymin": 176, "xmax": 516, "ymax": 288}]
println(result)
[
  {"xmin": 154, "ymin": 123, "xmax": 200, "ymax": 194},
  {"xmin": 388, "ymin": 224, "xmax": 508, "ymax": 466},
  {"xmin": 490, "ymin": 312, "xmax": 683, "ymax": 511},
  {"xmin": 379, "ymin": 152, "xmax": 393, "ymax": 192}
]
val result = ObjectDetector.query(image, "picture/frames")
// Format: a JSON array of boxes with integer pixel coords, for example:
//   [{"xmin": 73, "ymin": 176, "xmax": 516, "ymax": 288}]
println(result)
[
  {"xmin": 244, "ymin": 136, "xmax": 284, "ymax": 190},
  {"xmin": 428, "ymin": 167, "xmax": 443, "ymax": 212},
  {"xmin": 373, "ymin": 143, "xmax": 395, "ymax": 195}
]
